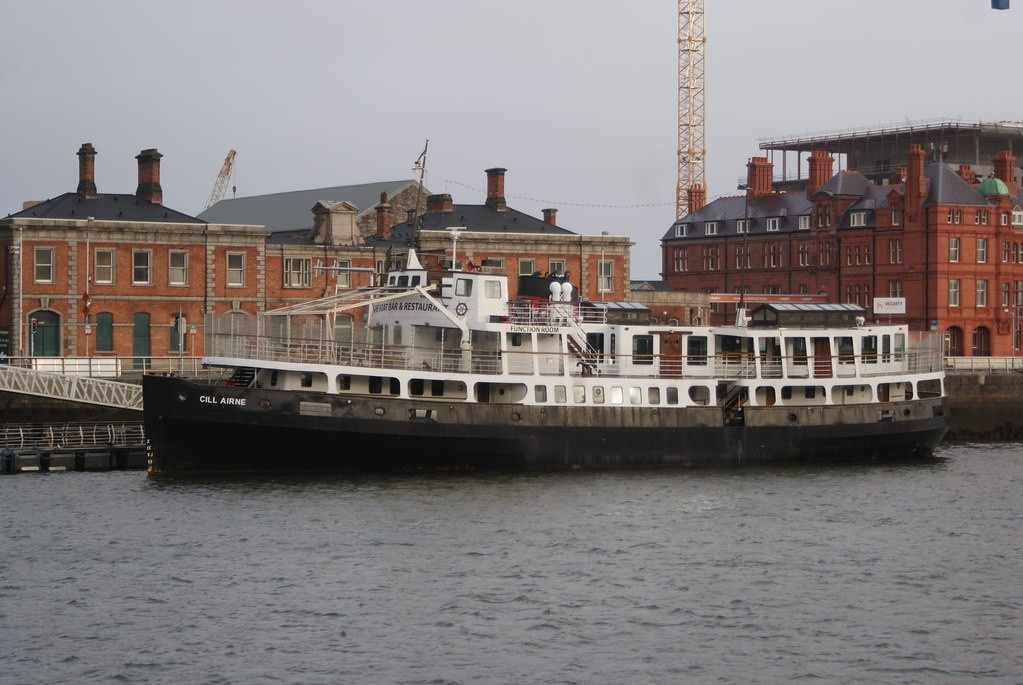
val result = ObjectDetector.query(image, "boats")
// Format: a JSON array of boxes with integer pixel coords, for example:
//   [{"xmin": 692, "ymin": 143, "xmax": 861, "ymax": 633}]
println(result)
[{"xmin": 139, "ymin": 223, "xmax": 953, "ymax": 471}]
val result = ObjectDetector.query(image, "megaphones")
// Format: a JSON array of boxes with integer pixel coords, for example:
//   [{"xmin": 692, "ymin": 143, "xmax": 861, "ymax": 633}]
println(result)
[
  {"xmin": 437, "ymin": 263, "xmax": 448, "ymax": 271},
  {"xmin": 467, "ymin": 261, "xmax": 481, "ymax": 272}
]
